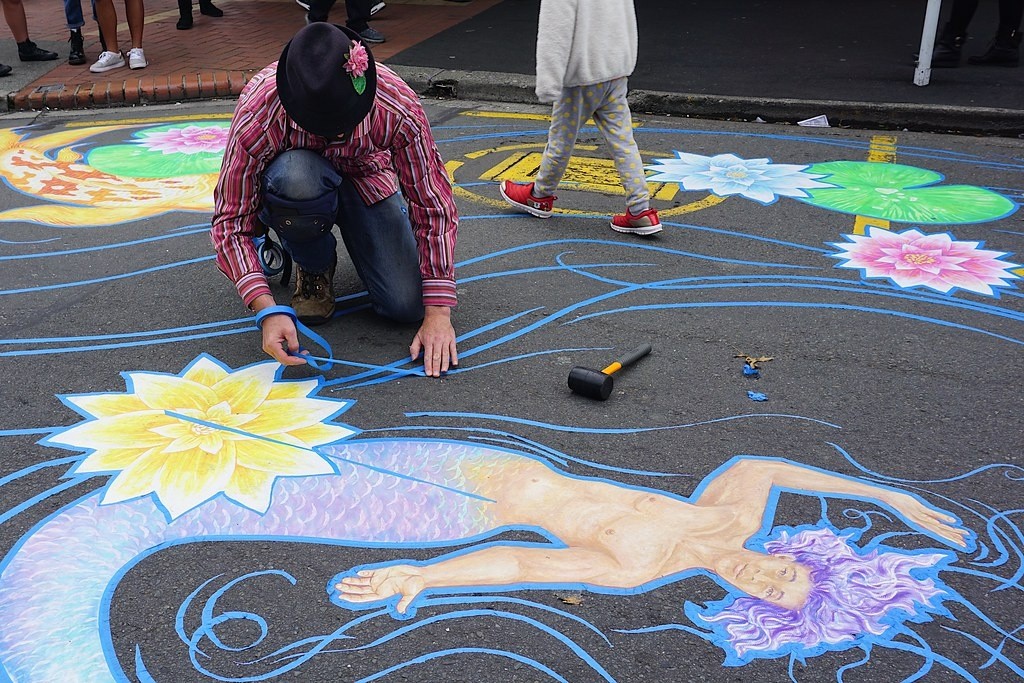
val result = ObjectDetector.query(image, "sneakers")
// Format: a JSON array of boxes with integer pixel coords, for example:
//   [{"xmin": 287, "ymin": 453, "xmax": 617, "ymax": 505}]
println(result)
[
  {"xmin": 357, "ymin": 26, "xmax": 385, "ymax": 43},
  {"xmin": 303, "ymin": 13, "xmax": 312, "ymax": 24},
  {"xmin": 89, "ymin": 49, "xmax": 126, "ymax": 73},
  {"xmin": 290, "ymin": 262, "xmax": 337, "ymax": 326},
  {"xmin": 126, "ymin": 47, "xmax": 147, "ymax": 69},
  {"xmin": 499, "ymin": 180, "xmax": 559, "ymax": 219},
  {"xmin": 609, "ymin": 206, "xmax": 663, "ymax": 236}
]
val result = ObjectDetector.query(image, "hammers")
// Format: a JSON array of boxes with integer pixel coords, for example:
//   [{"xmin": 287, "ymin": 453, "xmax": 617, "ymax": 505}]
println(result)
[{"xmin": 567, "ymin": 342, "xmax": 655, "ymax": 402}]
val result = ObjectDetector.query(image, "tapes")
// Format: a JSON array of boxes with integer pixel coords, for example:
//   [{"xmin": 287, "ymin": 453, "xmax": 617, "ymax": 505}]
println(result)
[{"xmin": 255, "ymin": 304, "xmax": 298, "ymax": 329}]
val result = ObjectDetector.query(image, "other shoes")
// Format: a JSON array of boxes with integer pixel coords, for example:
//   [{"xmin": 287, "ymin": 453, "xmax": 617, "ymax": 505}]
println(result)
[
  {"xmin": 294, "ymin": 0, "xmax": 311, "ymax": 10},
  {"xmin": 18, "ymin": 41, "xmax": 58, "ymax": 62},
  {"xmin": 0, "ymin": 64, "xmax": 12, "ymax": 76},
  {"xmin": 199, "ymin": 2, "xmax": 223, "ymax": 17},
  {"xmin": 176, "ymin": 16, "xmax": 194, "ymax": 30},
  {"xmin": 369, "ymin": 0, "xmax": 387, "ymax": 17}
]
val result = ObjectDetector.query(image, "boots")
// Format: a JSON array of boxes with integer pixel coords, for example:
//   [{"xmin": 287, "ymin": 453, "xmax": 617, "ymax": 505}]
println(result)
[{"xmin": 67, "ymin": 26, "xmax": 87, "ymax": 65}]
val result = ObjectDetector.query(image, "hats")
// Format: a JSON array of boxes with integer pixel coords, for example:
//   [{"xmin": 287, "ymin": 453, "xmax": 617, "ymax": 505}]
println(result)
[{"xmin": 275, "ymin": 21, "xmax": 377, "ymax": 142}]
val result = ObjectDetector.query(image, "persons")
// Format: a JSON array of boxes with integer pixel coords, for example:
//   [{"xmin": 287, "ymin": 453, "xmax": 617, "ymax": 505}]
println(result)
[
  {"xmin": 304, "ymin": 0, "xmax": 386, "ymax": 43},
  {"xmin": 210, "ymin": 22, "xmax": 459, "ymax": 378},
  {"xmin": 63, "ymin": 0, "xmax": 107, "ymax": 65},
  {"xmin": 90, "ymin": 0, "xmax": 147, "ymax": 72},
  {"xmin": 499, "ymin": 0, "xmax": 665, "ymax": 236},
  {"xmin": 176, "ymin": 0, "xmax": 223, "ymax": 30},
  {"xmin": 0, "ymin": 0, "xmax": 59, "ymax": 77}
]
